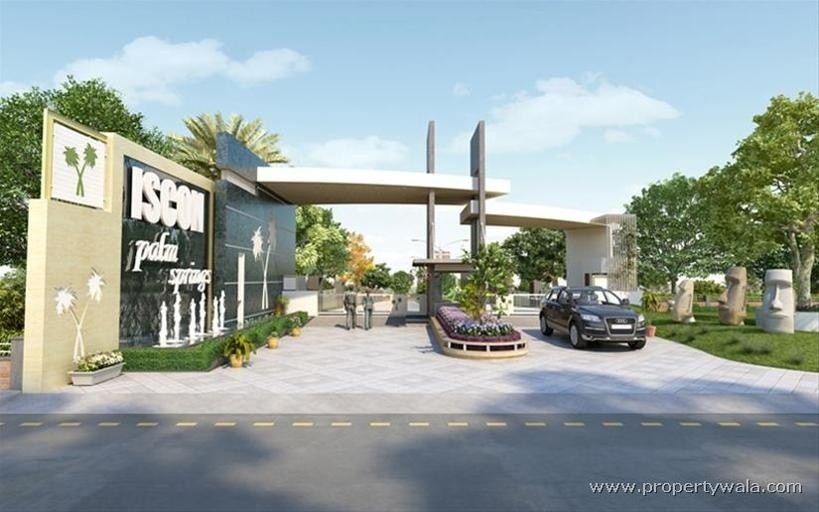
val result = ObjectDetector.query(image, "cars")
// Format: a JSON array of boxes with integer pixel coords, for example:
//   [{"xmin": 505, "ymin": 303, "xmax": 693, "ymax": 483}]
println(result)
[{"xmin": 539, "ymin": 286, "xmax": 647, "ymax": 350}]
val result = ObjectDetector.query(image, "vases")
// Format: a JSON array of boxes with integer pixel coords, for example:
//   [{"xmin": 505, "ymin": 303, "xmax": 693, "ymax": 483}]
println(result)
[{"xmin": 66, "ymin": 362, "xmax": 125, "ymax": 386}]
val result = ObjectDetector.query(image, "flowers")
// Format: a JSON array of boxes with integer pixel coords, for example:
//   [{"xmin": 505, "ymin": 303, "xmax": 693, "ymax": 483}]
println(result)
[{"xmin": 73, "ymin": 350, "xmax": 123, "ymax": 370}]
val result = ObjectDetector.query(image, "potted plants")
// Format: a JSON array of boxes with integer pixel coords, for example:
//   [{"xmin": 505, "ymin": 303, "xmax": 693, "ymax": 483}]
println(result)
[
  {"xmin": 290, "ymin": 313, "xmax": 302, "ymax": 337},
  {"xmin": 640, "ymin": 290, "xmax": 672, "ymax": 312},
  {"xmin": 267, "ymin": 331, "xmax": 279, "ymax": 348},
  {"xmin": 275, "ymin": 294, "xmax": 289, "ymax": 314},
  {"xmin": 646, "ymin": 319, "xmax": 656, "ymax": 337},
  {"xmin": 224, "ymin": 331, "xmax": 256, "ymax": 366}
]
